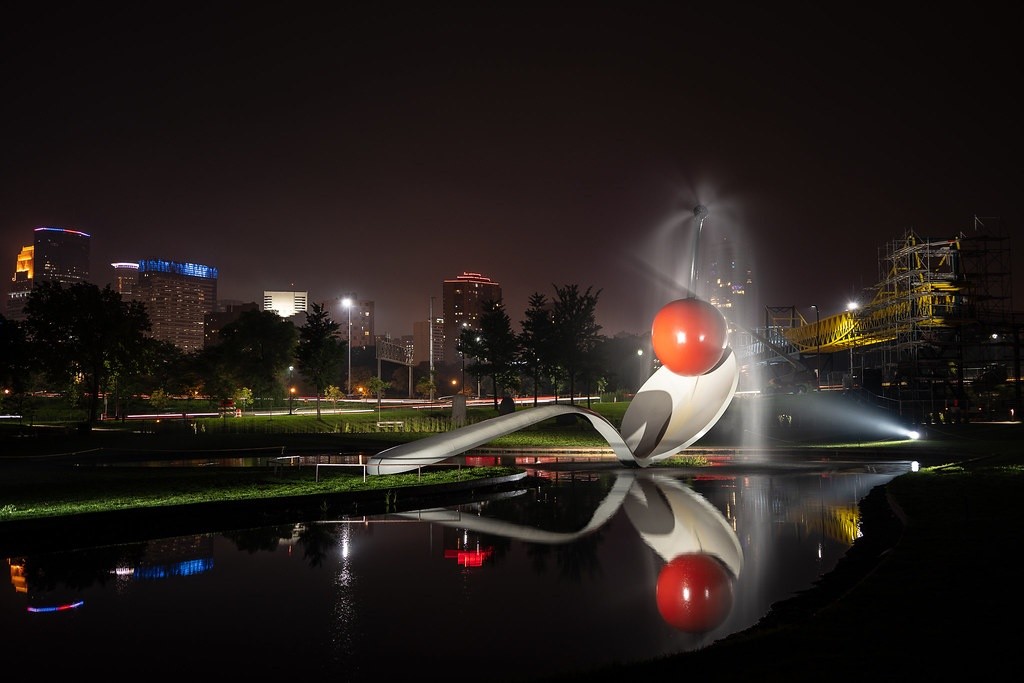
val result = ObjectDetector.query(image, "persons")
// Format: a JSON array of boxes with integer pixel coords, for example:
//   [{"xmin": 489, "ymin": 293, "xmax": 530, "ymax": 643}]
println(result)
[{"xmin": 946, "ymin": 362, "xmax": 957, "ymax": 377}]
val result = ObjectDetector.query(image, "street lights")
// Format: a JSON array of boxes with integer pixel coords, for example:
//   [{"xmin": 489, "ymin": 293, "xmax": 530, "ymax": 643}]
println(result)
[
  {"xmin": 811, "ymin": 305, "xmax": 820, "ymax": 391},
  {"xmin": 288, "ymin": 366, "xmax": 294, "ymax": 415},
  {"xmin": 343, "ymin": 298, "xmax": 353, "ymax": 397}
]
